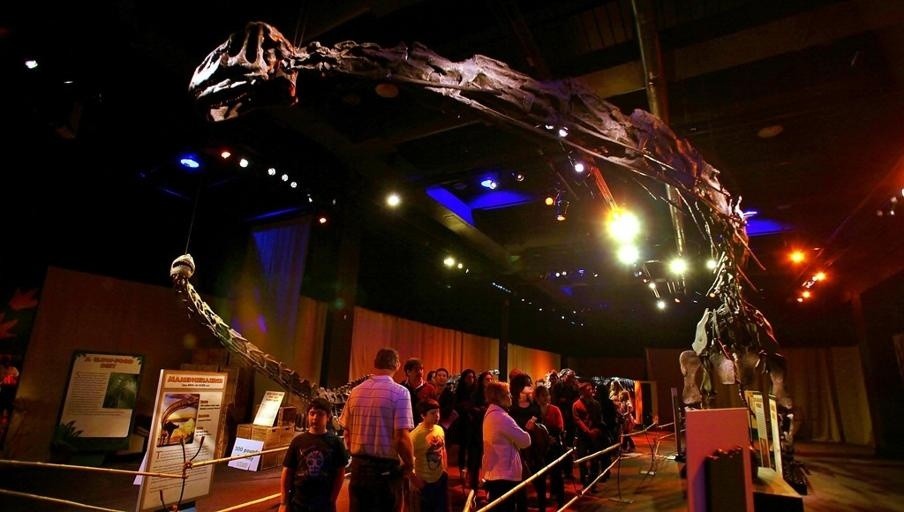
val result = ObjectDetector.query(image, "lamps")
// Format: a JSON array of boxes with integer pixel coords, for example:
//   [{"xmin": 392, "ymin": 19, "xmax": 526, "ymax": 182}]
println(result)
[{"xmin": 544, "ymin": 187, "xmax": 570, "ymax": 221}]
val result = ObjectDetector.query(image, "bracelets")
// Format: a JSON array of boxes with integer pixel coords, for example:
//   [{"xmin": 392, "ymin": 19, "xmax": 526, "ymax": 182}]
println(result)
[{"xmin": 281, "ymin": 502, "xmax": 288, "ymax": 505}]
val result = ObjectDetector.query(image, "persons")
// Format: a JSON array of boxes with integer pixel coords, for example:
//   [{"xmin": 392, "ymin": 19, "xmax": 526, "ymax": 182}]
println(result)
[
  {"xmin": 398, "ymin": 358, "xmax": 637, "ymax": 511},
  {"xmin": 278, "ymin": 398, "xmax": 349, "ymax": 511},
  {"xmin": 338, "ymin": 350, "xmax": 414, "ymax": 511},
  {"xmin": 0, "ymin": 355, "xmax": 20, "ymax": 421}
]
